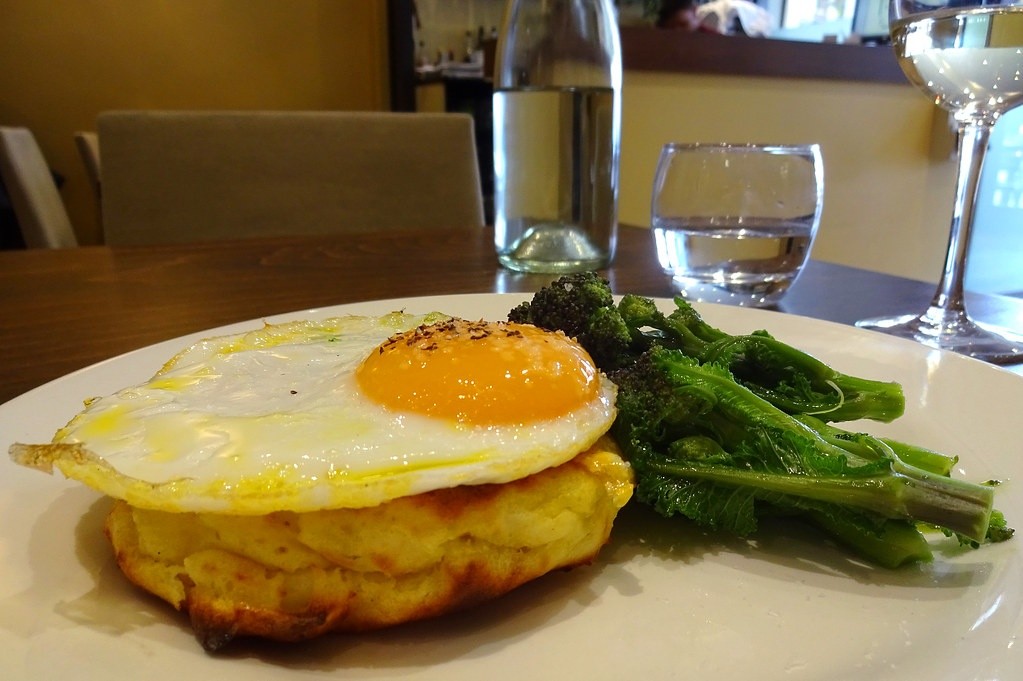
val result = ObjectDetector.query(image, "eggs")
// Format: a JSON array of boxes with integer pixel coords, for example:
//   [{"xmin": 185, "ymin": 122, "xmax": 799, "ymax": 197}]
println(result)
[{"xmin": 9, "ymin": 308, "xmax": 619, "ymax": 514}]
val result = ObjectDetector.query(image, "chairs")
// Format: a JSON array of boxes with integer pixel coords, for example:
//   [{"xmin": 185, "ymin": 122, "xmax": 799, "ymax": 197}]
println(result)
[{"xmin": 96, "ymin": 109, "xmax": 485, "ymax": 246}]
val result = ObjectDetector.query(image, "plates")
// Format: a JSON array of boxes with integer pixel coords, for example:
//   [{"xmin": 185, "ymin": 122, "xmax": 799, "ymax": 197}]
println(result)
[{"xmin": 0, "ymin": 292, "xmax": 1023, "ymax": 681}]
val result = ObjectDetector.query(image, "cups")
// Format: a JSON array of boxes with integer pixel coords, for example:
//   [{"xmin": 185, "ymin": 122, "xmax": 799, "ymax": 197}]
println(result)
[{"xmin": 650, "ymin": 139, "xmax": 823, "ymax": 308}]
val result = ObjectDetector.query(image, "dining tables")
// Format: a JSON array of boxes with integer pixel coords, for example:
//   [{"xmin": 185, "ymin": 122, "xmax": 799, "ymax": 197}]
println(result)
[{"xmin": 0, "ymin": 214, "xmax": 1023, "ymax": 681}]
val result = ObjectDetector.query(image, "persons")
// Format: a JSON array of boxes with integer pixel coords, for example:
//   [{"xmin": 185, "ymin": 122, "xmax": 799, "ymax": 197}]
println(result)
[{"xmin": 656, "ymin": 0, "xmax": 719, "ymax": 33}]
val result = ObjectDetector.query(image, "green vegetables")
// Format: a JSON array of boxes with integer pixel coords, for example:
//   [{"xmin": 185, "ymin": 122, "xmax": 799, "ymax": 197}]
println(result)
[{"xmin": 504, "ymin": 270, "xmax": 1017, "ymax": 570}]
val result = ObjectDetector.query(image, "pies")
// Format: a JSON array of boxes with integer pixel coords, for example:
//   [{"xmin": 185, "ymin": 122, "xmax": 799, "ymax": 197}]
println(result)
[{"xmin": 106, "ymin": 436, "xmax": 634, "ymax": 639}]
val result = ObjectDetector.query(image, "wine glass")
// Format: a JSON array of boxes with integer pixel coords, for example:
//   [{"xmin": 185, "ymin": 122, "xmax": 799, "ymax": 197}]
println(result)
[{"xmin": 854, "ymin": 0, "xmax": 1023, "ymax": 367}]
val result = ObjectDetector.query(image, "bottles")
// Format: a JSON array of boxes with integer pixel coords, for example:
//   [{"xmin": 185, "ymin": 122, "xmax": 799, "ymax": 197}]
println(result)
[{"xmin": 493, "ymin": 0, "xmax": 625, "ymax": 276}]
